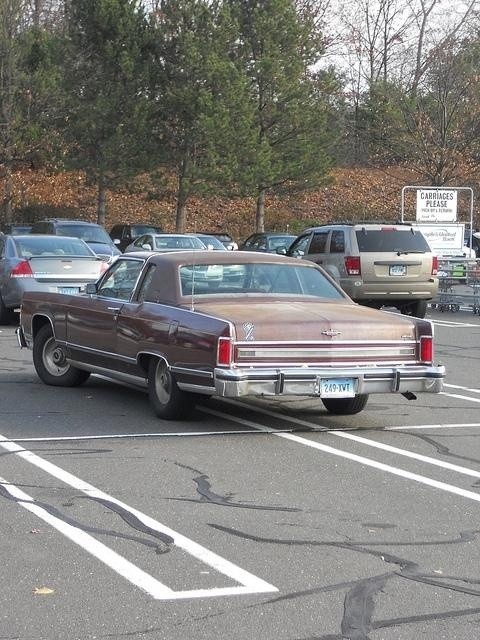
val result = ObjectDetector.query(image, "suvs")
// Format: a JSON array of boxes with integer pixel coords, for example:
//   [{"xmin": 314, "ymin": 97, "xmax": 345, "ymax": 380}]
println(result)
[{"xmin": 276, "ymin": 222, "xmax": 438, "ymax": 319}]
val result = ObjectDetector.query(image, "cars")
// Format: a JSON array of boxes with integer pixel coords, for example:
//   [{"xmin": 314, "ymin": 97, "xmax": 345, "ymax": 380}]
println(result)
[
  {"xmin": 1, "ymin": 218, "xmax": 239, "ymax": 325},
  {"xmin": 16, "ymin": 249, "xmax": 447, "ymax": 421},
  {"xmin": 241, "ymin": 233, "xmax": 299, "ymax": 256}
]
